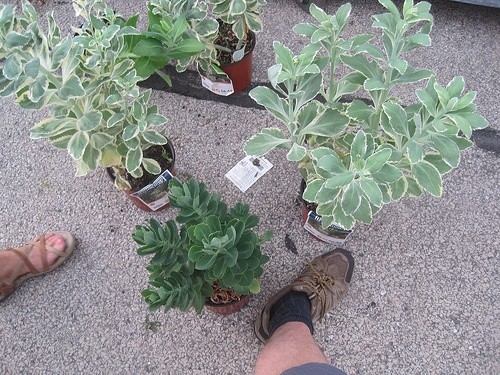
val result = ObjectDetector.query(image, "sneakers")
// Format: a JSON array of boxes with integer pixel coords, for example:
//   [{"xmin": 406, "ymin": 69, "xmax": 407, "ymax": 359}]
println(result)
[{"xmin": 255, "ymin": 247, "xmax": 356, "ymax": 346}]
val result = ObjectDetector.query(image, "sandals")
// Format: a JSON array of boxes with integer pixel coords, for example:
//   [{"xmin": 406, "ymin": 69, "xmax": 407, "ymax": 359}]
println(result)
[{"xmin": 0, "ymin": 230, "xmax": 75, "ymax": 302}]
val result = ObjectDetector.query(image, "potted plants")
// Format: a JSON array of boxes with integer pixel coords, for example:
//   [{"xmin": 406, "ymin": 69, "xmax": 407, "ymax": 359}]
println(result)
[
  {"xmin": 0, "ymin": 0, "xmax": 177, "ymax": 213},
  {"xmin": 133, "ymin": 177, "xmax": 274, "ymax": 315},
  {"xmin": 243, "ymin": 0, "xmax": 489, "ymax": 245},
  {"xmin": 146, "ymin": 0, "xmax": 267, "ymax": 96}
]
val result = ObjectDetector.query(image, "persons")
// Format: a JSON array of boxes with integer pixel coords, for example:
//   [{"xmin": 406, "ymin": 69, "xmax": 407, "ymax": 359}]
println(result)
[
  {"xmin": 253, "ymin": 248, "xmax": 356, "ymax": 375},
  {"xmin": 0, "ymin": 230, "xmax": 76, "ymax": 301}
]
